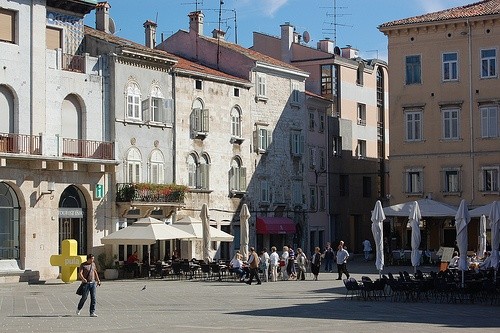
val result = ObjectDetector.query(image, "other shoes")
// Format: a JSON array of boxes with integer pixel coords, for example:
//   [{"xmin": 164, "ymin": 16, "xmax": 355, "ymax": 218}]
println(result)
[
  {"xmin": 255, "ymin": 282, "xmax": 261, "ymax": 285},
  {"xmin": 347, "ymin": 275, "xmax": 350, "ymax": 280},
  {"xmin": 90, "ymin": 312, "xmax": 98, "ymax": 317},
  {"xmin": 244, "ymin": 280, "xmax": 251, "ymax": 285},
  {"xmin": 336, "ymin": 277, "xmax": 342, "ymax": 280},
  {"xmin": 76, "ymin": 307, "xmax": 81, "ymax": 315}
]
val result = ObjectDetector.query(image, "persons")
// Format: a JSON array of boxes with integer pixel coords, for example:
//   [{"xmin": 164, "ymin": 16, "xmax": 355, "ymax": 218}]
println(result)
[
  {"xmin": 311, "ymin": 247, "xmax": 321, "ymax": 280},
  {"xmin": 232, "ymin": 250, "xmax": 243, "ymax": 273},
  {"xmin": 340, "ymin": 241, "xmax": 347, "ymax": 250},
  {"xmin": 336, "ymin": 245, "xmax": 350, "ymax": 280},
  {"xmin": 363, "ymin": 239, "xmax": 372, "ymax": 262},
  {"xmin": 76, "ymin": 254, "xmax": 101, "ymax": 317},
  {"xmin": 127, "ymin": 252, "xmax": 140, "ymax": 276},
  {"xmin": 269, "ymin": 246, "xmax": 306, "ymax": 282},
  {"xmin": 260, "ymin": 248, "xmax": 269, "ymax": 282},
  {"xmin": 245, "ymin": 247, "xmax": 261, "ymax": 285},
  {"xmin": 172, "ymin": 251, "xmax": 179, "ymax": 261},
  {"xmin": 324, "ymin": 242, "xmax": 335, "ymax": 272}
]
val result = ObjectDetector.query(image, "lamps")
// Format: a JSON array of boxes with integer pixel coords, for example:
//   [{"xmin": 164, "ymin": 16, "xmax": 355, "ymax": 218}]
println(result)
[{"xmin": 42, "ymin": 180, "xmax": 55, "ymax": 194}]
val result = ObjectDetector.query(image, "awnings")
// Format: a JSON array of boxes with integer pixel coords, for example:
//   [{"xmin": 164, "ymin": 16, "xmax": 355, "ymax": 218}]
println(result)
[{"xmin": 256, "ymin": 217, "xmax": 296, "ymax": 235}]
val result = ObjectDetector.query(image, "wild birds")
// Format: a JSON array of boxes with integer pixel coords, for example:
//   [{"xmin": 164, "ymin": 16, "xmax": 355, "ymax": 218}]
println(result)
[{"xmin": 142, "ymin": 285, "xmax": 146, "ymax": 290}]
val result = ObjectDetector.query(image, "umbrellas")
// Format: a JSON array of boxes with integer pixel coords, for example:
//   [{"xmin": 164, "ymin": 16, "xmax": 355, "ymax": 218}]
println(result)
[
  {"xmin": 479, "ymin": 214, "xmax": 486, "ymax": 259},
  {"xmin": 372, "ymin": 198, "xmax": 458, "ymax": 217},
  {"xmin": 409, "ymin": 201, "xmax": 422, "ymax": 273},
  {"xmin": 168, "ymin": 216, "xmax": 234, "ymax": 241},
  {"xmin": 468, "ymin": 202, "xmax": 492, "ymax": 217},
  {"xmin": 199, "ymin": 203, "xmax": 211, "ymax": 264},
  {"xmin": 454, "ymin": 199, "xmax": 471, "ymax": 283},
  {"xmin": 239, "ymin": 204, "xmax": 251, "ymax": 260},
  {"xmin": 481, "ymin": 201, "xmax": 500, "ymax": 270},
  {"xmin": 371, "ymin": 200, "xmax": 386, "ymax": 280},
  {"xmin": 101, "ymin": 216, "xmax": 197, "ymax": 277}
]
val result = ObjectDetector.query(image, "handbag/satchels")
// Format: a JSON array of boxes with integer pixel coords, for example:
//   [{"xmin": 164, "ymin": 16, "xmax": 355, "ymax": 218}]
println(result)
[{"xmin": 76, "ymin": 282, "xmax": 86, "ymax": 295}]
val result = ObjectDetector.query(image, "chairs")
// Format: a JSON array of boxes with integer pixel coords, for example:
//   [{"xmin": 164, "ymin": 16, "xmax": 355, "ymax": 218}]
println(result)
[
  {"xmin": 115, "ymin": 258, "xmax": 237, "ymax": 282},
  {"xmin": 343, "ymin": 267, "xmax": 500, "ymax": 304}
]
